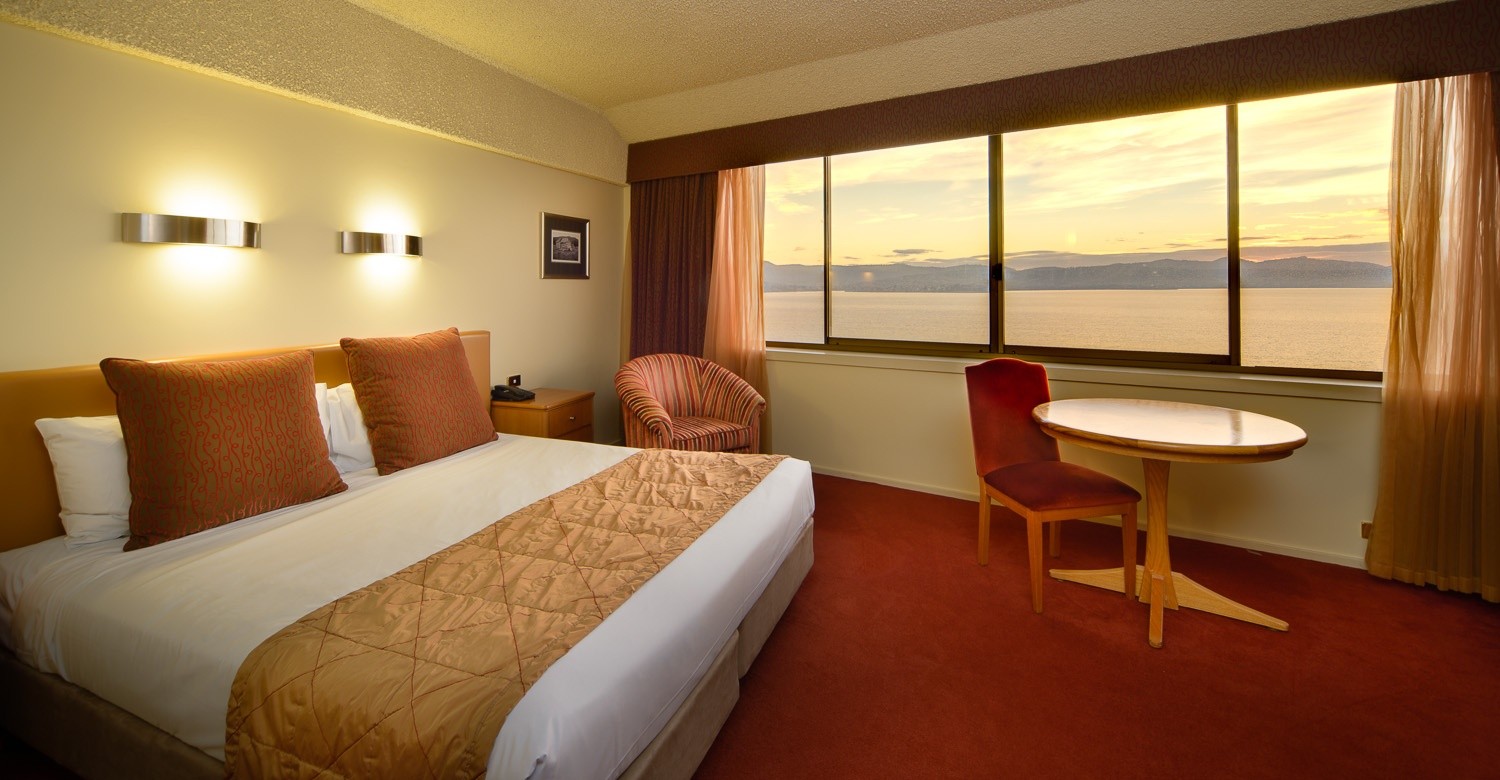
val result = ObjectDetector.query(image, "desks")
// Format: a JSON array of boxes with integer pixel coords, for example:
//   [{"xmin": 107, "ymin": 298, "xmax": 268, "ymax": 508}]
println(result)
[{"xmin": 1034, "ymin": 397, "xmax": 1305, "ymax": 646}]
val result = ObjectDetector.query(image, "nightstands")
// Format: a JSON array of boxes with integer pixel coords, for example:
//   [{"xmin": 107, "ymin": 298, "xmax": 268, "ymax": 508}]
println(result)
[{"xmin": 491, "ymin": 388, "xmax": 595, "ymax": 447}]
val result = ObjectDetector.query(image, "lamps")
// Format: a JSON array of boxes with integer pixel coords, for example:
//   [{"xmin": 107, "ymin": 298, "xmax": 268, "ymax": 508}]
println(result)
[
  {"xmin": 342, "ymin": 230, "xmax": 423, "ymax": 257},
  {"xmin": 122, "ymin": 212, "xmax": 260, "ymax": 250}
]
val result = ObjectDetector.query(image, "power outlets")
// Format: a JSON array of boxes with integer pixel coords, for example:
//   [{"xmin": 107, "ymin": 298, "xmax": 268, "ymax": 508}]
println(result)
[
  {"xmin": 1361, "ymin": 520, "xmax": 1373, "ymax": 540},
  {"xmin": 507, "ymin": 373, "xmax": 521, "ymax": 387}
]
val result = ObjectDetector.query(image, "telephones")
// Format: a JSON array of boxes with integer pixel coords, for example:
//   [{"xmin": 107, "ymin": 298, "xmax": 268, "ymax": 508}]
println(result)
[{"xmin": 491, "ymin": 384, "xmax": 536, "ymax": 401}]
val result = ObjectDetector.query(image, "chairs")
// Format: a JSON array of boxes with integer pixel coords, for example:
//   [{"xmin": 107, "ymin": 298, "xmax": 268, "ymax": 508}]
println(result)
[
  {"xmin": 615, "ymin": 354, "xmax": 765, "ymax": 460},
  {"xmin": 963, "ymin": 356, "xmax": 1141, "ymax": 611}
]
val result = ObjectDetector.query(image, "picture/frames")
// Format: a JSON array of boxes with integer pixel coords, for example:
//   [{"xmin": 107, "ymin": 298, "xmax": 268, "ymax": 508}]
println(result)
[{"xmin": 540, "ymin": 212, "xmax": 591, "ymax": 282}]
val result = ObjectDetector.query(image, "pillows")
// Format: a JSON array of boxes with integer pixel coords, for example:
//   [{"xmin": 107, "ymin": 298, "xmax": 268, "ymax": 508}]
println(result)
[
  {"xmin": 326, "ymin": 385, "xmax": 375, "ymax": 476},
  {"xmin": 98, "ymin": 352, "xmax": 348, "ymax": 551},
  {"xmin": 35, "ymin": 417, "xmax": 132, "ymax": 543},
  {"xmin": 316, "ymin": 382, "xmax": 332, "ymax": 457},
  {"xmin": 341, "ymin": 326, "xmax": 499, "ymax": 479}
]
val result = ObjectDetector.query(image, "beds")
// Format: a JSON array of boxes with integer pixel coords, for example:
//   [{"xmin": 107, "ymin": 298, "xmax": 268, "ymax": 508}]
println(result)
[{"xmin": 0, "ymin": 333, "xmax": 816, "ymax": 780}]
